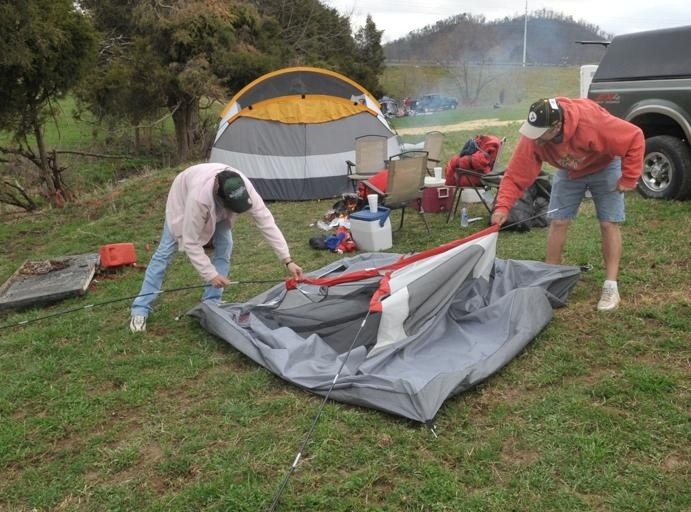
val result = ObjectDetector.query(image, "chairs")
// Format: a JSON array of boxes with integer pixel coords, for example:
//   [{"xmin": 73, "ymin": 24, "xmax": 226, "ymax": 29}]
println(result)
[{"xmin": 341, "ymin": 130, "xmax": 510, "ymax": 253}]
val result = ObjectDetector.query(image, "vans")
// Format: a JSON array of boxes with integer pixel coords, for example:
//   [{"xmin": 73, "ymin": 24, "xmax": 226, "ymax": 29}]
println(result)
[{"xmin": 573, "ymin": 26, "xmax": 691, "ymax": 205}]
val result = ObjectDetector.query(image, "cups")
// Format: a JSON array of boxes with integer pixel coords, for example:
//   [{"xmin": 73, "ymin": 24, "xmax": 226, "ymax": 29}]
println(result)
[
  {"xmin": 434, "ymin": 167, "xmax": 443, "ymax": 180},
  {"xmin": 366, "ymin": 194, "xmax": 379, "ymax": 214}
]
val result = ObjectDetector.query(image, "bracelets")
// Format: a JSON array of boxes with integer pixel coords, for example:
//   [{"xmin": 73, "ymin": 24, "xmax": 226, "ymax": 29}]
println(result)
[{"xmin": 286, "ymin": 260, "xmax": 293, "ymax": 265}]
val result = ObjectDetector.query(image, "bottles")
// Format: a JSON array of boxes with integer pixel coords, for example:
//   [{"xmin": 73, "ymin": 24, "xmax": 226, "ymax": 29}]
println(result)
[{"xmin": 461, "ymin": 206, "xmax": 470, "ymax": 227}]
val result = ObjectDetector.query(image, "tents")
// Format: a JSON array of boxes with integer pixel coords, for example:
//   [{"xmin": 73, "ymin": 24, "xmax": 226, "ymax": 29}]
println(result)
[
  {"xmin": 378, "ymin": 95, "xmax": 409, "ymax": 118},
  {"xmin": 176, "ymin": 225, "xmax": 592, "ymax": 437},
  {"xmin": 206, "ymin": 65, "xmax": 403, "ymax": 202}
]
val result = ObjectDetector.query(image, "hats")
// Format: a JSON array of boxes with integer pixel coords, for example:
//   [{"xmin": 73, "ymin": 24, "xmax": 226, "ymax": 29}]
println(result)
[
  {"xmin": 219, "ymin": 171, "xmax": 253, "ymax": 213},
  {"xmin": 518, "ymin": 98, "xmax": 564, "ymax": 141}
]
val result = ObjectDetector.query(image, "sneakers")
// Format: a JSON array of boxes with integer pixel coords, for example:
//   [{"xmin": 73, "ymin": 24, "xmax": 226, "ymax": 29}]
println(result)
[
  {"xmin": 130, "ymin": 316, "xmax": 147, "ymax": 334},
  {"xmin": 597, "ymin": 288, "xmax": 621, "ymax": 309}
]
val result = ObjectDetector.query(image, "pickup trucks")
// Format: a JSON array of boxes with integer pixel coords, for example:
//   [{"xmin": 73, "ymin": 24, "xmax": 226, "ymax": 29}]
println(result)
[{"xmin": 414, "ymin": 94, "xmax": 461, "ymax": 116}]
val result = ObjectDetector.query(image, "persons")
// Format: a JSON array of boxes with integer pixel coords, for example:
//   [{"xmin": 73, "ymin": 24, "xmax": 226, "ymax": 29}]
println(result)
[
  {"xmin": 129, "ymin": 164, "xmax": 304, "ymax": 334},
  {"xmin": 491, "ymin": 97, "xmax": 646, "ymax": 311},
  {"xmin": 405, "ymin": 97, "xmax": 413, "ymax": 110}
]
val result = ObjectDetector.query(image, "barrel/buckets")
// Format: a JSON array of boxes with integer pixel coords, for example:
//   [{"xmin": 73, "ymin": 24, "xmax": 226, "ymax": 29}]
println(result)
[
  {"xmin": 99, "ymin": 242, "xmax": 137, "ymax": 268},
  {"xmin": 460, "ymin": 187, "xmax": 486, "ymax": 203}
]
